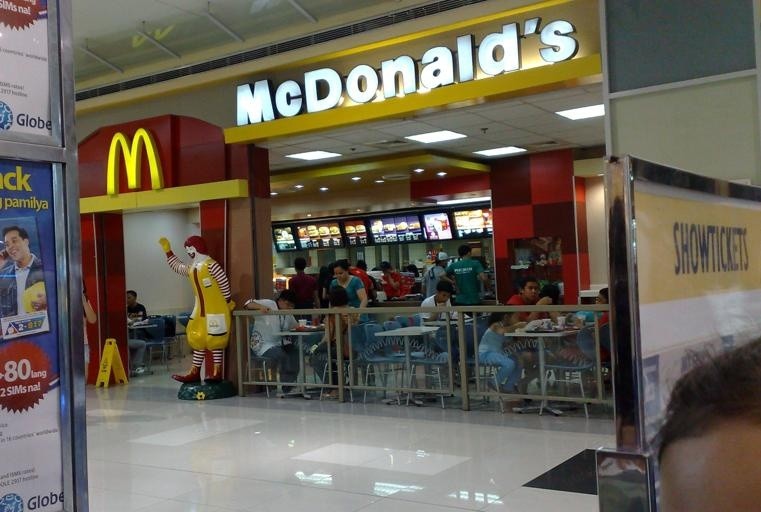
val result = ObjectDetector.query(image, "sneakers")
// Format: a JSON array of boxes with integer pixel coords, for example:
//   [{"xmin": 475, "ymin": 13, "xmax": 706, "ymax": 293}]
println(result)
[
  {"xmin": 319, "ymin": 389, "xmax": 339, "ymax": 399},
  {"xmin": 134, "ymin": 366, "xmax": 145, "ymax": 374}
]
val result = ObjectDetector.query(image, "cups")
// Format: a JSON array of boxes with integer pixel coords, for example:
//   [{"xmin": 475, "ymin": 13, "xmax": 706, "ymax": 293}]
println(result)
[
  {"xmin": 576, "ymin": 314, "xmax": 587, "ymax": 328},
  {"xmin": 543, "ymin": 318, "xmax": 552, "ymax": 330},
  {"xmin": 313, "ymin": 313, "xmax": 320, "ymax": 325},
  {"xmin": 299, "ymin": 320, "xmax": 307, "ymax": 327},
  {"xmin": 558, "ymin": 317, "xmax": 567, "ymax": 329}
]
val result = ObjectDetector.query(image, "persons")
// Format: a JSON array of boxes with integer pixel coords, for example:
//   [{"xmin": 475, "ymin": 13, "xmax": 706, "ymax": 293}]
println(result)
[
  {"xmin": 0, "ymin": 226, "xmax": 46, "ymax": 319},
  {"xmin": 130, "ymin": 339, "xmax": 152, "ymax": 374},
  {"xmin": 244, "ymin": 244, "xmax": 610, "ymax": 409},
  {"xmin": 128, "ymin": 289, "xmax": 146, "ymax": 323},
  {"xmin": 157, "ymin": 235, "xmax": 237, "ymax": 383},
  {"xmin": 83, "ymin": 282, "xmax": 97, "ymax": 383},
  {"xmin": 657, "ymin": 337, "xmax": 760, "ymax": 511}
]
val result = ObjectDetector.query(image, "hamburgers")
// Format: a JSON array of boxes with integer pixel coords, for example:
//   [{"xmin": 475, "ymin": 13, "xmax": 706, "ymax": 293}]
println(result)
[{"xmin": 308, "ymin": 225, "xmax": 365, "ymax": 236}]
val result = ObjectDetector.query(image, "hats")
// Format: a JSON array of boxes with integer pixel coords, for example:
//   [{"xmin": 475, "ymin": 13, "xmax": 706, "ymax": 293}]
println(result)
[{"xmin": 436, "ymin": 252, "xmax": 450, "ymax": 262}]
